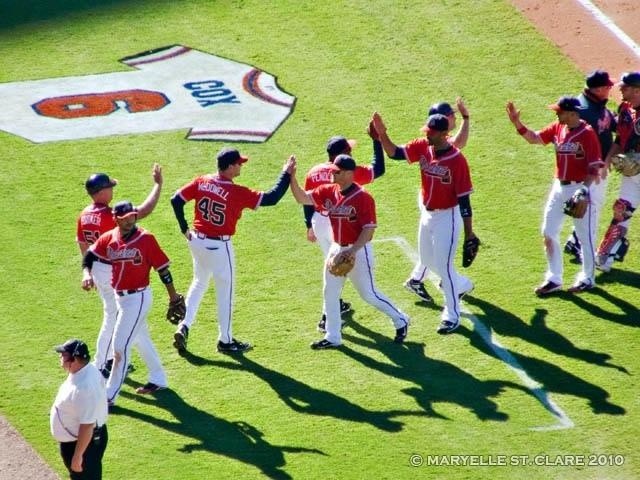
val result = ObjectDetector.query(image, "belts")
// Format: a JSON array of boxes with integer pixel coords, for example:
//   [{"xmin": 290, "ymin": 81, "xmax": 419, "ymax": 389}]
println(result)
[
  {"xmin": 116, "ymin": 289, "xmax": 137, "ymax": 296},
  {"xmin": 335, "ymin": 242, "xmax": 350, "ymax": 248},
  {"xmin": 426, "ymin": 207, "xmax": 445, "ymax": 212},
  {"xmin": 559, "ymin": 181, "xmax": 582, "ymax": 186},
  {"xmin": 94, "ymin": 258, "xmax": 112, "ymax": 265},
  {"xmin": 195, "ymin": 231, "xmax": 221, "ymax": 241}
]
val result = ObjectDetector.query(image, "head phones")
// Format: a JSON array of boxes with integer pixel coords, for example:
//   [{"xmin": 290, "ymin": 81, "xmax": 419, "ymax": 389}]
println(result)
[{"xmin": 71, "ymin": 340, "xmax": 81, "ymax": 363}]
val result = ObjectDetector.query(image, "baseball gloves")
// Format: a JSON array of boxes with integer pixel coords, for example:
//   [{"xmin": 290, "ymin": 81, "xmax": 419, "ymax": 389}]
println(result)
[
  {"xmin": 563, "ymin": 190, "xmax": 589, "ymax": 218},
  {"xmin": 611, "ymin": 155, "xmax": 639, "ymax": 177},
  {"xmin": 327, "ymin": 251, "xmax": 355, "ymax": 275},
  {"xmin": 463, "ymin": 237, "xmax": 480, "ymax": 266},
  {"xmin": 167, "ymin": 294, "xmax": 186, "ymax": 325}
]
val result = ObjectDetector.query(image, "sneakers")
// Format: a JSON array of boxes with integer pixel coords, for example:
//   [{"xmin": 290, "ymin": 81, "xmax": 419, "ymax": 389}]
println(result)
[
  {"xmin": 403, "ymin": 278, "xmax": 474, "ymax": 335},
  {"xmin": 534, "ymin": 240, "xmax": 610, "ymax": 295},
  {"xmin": 99, "ymin": 359, "xmax": 160, "ymax": 404},
  {"xmin": 217, "ymin": 337, "xmax": 250, "ymax": 352},
  {"xmin": 394, "ymin": 314, "xmax": 411, "ymax": 343},
  {"xmin": 310, "ymin": 339, "xmax": 342, "ymax": 351},
  {"xmin": 173, "ymin": 327, "xmax": 187, "ymax": 350}
]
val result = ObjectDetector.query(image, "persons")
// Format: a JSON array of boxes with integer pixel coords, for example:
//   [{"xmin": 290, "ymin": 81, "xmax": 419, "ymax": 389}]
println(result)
[
  {"xmin": 505, "ymin": 94, "xmax": 609, "ymax": 298},
  {"xmin": 80, "ymin": 199, "xmax": 186, "ymax": 407},
  {"xmin": 290, "ymin": 97, "xmax": 482, "ymax": 350},
  {"xmin": 48, "ymin": 338, "xmax": 108, "ymax": 479},
  {"xmin": 172, "ymin": 145, "xmax": 296, "ymax": 355},
  {"xmin": 564, "ymin": 69, "xmax": 639, "ymax": 273},
  {"xmin": 76, "ymin": 162, "xmax": 164, "ymax": 377}
]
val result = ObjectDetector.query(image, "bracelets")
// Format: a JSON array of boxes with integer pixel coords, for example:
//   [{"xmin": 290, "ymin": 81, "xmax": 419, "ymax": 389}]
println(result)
[
  {"xmin": 159, "ymin": 272, "xmax": 173, "ymax": 284},
  {"xmin": 515, "ymin": 125, "xmax": 527, "ymax": 136}
]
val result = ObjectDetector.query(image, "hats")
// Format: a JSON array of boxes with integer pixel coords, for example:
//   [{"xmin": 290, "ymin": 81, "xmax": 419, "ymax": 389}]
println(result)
[
  {"xmin": 217, "ymin": 148, "xmax": 248, "ymax": 167},
  {"xmin": 324, "ymin": 99, "xmax": 459, "ymax": 171},
  {"xmin": 54, "ymin": 339, "xmax": 89, "ymax": 358},
  {"xmin": 318, "ymin": 299, "xmax": 351, "ymax": 332},
  {"xmin": 547, "ymin": 71, "xmax": 640, "ymax": 112},
  {"xmin": 85, "ymin": 173, "xmax": 139, "ymax": 218}
]
